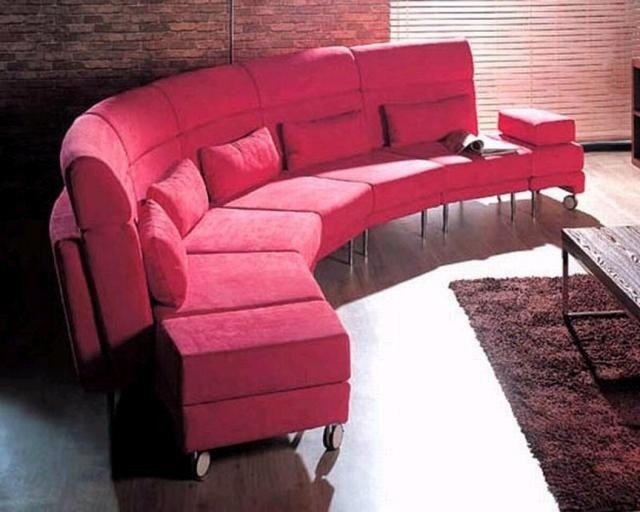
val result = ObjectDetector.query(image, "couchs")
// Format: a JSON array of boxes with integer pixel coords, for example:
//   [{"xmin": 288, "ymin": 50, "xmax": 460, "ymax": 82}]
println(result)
[{"xmin": 31, "ymin": 33, "xmax": 586, "ymax": 479}]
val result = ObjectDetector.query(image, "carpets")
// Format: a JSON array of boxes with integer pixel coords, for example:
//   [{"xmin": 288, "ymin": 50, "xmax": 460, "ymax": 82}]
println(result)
[{"xmin": 446, "ymin": 272, "xmax": 640, "ymax": 512}]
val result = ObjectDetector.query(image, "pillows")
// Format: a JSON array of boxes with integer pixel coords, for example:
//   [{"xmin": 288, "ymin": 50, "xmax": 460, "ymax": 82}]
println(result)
[
  {"xmin": 279, "ymin": 110, "xmax": 372, "ymax": 172},
  {"xmin": 381, "ymin": 94, "xmax": 477, "ymax": 149},
  {"xmin": 140, "ymin": 198, "xmax": 190, "ymax": 307},
  {"xmin": 149, "ymin": 155, "xmax": 211, "ymax": 237},
  {"xmin": 202, "ymin": 123, "xmax": 283, "ymax": 207}
]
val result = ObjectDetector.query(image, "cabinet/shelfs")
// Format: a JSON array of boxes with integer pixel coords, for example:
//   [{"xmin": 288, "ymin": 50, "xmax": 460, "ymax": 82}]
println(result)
[{"xmin": 630, "ymin": 56, "xmax": 640, "ymax": 168}]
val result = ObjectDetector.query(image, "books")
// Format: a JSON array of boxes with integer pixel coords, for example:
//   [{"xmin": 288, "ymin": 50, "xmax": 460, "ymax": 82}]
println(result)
[{"xmin": 438, "ymin": 128, "xmax": 518, "ymax": 156}]
